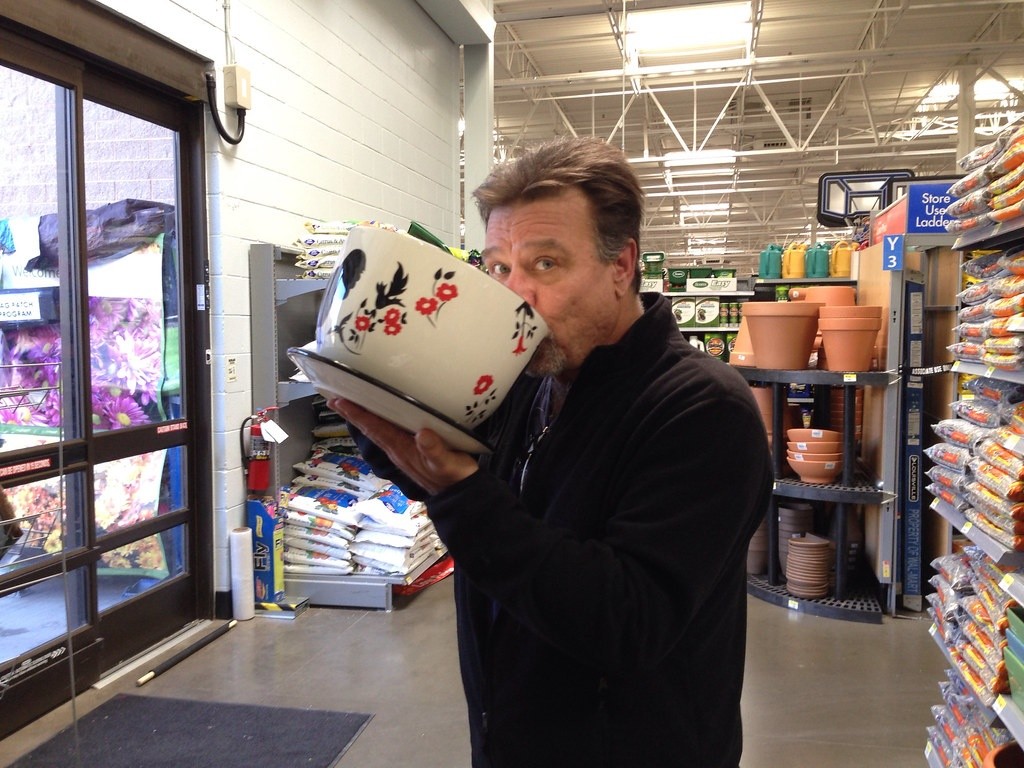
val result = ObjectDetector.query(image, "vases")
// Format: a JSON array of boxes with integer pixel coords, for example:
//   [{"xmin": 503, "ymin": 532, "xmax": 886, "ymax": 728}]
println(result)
[
  {"xmin": 290, "ymin": 226, "xmax": 552, "ymax": 458},
  {"xmin": 727, "ymin": 284, "xmax": 884, "ymax": 374}
]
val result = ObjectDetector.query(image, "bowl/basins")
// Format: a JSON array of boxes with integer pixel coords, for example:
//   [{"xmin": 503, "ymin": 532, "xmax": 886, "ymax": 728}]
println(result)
[
  {"xmin": 747, "ymin": 501, "xmax": 814, "ymax": 576},
  {"xmin": 785, "ymin": 429, "xmax": 844, "ymax": 483},
  {"xmin": 315, "ymin": 224, "xmax": 550, "ymax": 428}
]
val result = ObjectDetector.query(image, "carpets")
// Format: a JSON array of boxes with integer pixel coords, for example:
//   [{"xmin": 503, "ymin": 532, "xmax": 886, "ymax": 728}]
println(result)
[{"xmin": 12, "ymin": 692, "xmax": 376, "ymax": 767}]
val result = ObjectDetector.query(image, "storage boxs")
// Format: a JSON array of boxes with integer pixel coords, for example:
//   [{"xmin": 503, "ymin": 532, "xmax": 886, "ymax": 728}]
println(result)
[{"xmin": 638, "ymin": 250, "xmax": 738, "ymax": 328}]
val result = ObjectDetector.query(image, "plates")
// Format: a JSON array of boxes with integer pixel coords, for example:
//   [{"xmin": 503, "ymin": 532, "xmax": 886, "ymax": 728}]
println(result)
[{"xmin": 785, "ymin": 537, "xmax": 830, "ymax": 598}]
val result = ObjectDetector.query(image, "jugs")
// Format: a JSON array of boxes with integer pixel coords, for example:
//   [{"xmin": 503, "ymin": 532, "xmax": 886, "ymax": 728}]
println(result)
[{"xmin": 760, "ymin": 242, "xmax": 859, "ymax": 277}]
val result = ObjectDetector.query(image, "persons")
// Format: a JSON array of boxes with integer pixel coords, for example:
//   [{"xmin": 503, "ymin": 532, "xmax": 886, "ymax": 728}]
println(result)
[{"xmin": 327, "ymin": 138, "xmax": 775, "ymax": 768}]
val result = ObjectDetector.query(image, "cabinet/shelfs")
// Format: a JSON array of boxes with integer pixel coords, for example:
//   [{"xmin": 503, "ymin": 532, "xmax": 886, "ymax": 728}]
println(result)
[
  {"xmin": 642, "ymin": 277, "xmax": 859, "ymax": 365},
  {"xmin": 925, "ymin": 215, "xmax": 1023, "ymax": 768},
  {"xmin": 248, "ymin": 242, "xmax": 395, "ymax": 612},
  {"xmin": 652, "ymin": 368, "xmax": 896, "ymax": 624}
]
